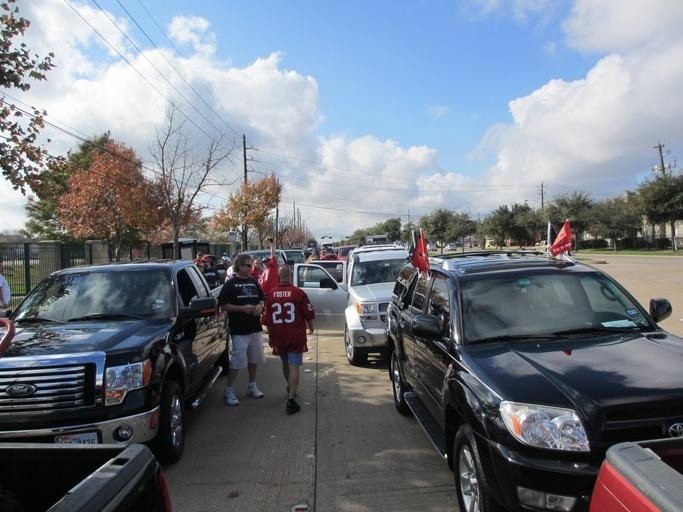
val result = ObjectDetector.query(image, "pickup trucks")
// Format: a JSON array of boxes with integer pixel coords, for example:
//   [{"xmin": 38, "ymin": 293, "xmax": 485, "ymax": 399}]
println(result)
[
  {"xmin": 0, "ymin": 443, "xmax": 173, "ymax": 512},
  {"xmin": 1, "ymin": 258, "xmax": 232, "ymax": 463},
  {"xmin": 589, "ymin": 435, "xmax": 683, "ymax": 511}
]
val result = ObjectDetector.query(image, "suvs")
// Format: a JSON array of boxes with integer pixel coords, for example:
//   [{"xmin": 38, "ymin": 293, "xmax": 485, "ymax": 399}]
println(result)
[
  {"xmin": 386, "ymin": 252, "xmax": 682, "ymax": 512},
  {"xmin": 199, "ymin": 232, "xmax": 410, "ymax": 365}
]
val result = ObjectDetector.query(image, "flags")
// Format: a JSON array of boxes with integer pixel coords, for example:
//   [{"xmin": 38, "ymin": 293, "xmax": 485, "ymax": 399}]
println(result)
[
  {"xmin": 411, "ymin": 231, "xmax": 431, "ymax": 276},
  {"xmin": 548, "ymin": 218, "xmax": 572, "ymax": 256},
  {"xmin": 405, "ymin": 231, "xmax": 417, "ymax": 262}
]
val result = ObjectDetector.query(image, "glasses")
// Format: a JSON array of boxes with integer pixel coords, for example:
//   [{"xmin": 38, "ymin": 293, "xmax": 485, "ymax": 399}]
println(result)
[{"xmin": 244, "ymin": 263, "xmax": 252, "ymax": 268}]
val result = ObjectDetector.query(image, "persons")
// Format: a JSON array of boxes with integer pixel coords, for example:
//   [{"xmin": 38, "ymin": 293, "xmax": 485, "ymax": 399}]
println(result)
[
  {"xmin": 305, "ymin": 249, "xmax": 333, "ymax": 264},
  {"xmin": 219, "ymin": 254, "xmax": 266, "ymax": 405},
  {"xmin": 262, "ymin": 266, "xmax": 316, "ymax": 415},
  {"xmin": 192, "ymin": 251, "xmax": 279, "ymax": 333},
  {"xmin": 1, "ymin": 261, "xmax": 11, "ymax": 310}
]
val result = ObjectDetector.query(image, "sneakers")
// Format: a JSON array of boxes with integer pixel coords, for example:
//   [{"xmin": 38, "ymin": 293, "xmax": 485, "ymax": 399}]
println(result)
[
  {"xmin": 222, "ymin": 388, "xmax": 239, "ymax": 405},
  {"xmin": 246, "ymin": 384, "xmax": 264, "ymax": 398},
  {"xmin": 286, "ymin": 400, "xmax": 300, "ymax": 412}
]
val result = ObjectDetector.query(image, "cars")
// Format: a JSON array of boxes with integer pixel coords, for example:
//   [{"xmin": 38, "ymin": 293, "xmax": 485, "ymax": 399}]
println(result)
[{"xmin": 426, "ymin": 240, "xmax": 479, "ymax": 253}]
